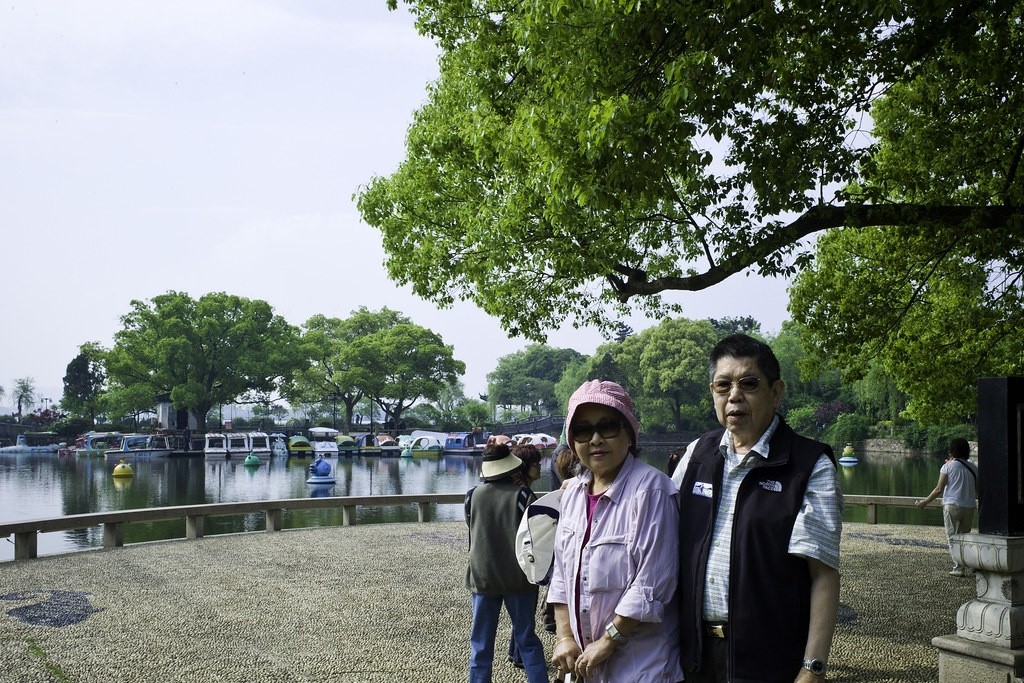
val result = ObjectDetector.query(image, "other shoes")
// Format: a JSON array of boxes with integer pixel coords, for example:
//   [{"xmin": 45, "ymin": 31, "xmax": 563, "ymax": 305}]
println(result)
[
  {"xmin": 948, "ymin": 570, "xmax": 964, "ymax": 576},
  {"xmin": 543, "ymin": 615, "xmax": 556, "ymax": 624},
  {"xmin": 508, "ymin": 655, "xmax": 524, "ymax": 668},
  {"xmin": 545, "ymin": 622, "xmax": 556, "ymax": 631}
]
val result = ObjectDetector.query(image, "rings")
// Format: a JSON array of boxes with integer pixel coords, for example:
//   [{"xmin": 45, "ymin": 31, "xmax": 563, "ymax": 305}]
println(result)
[{"xmin": 556, "ymin": 666, "xmax": 562, "ymax": 671}]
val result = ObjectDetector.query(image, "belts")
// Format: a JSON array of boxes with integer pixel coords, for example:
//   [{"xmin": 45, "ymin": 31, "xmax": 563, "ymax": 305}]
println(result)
[{"xmin": 703, "ymin": 624, "xmax": 728, "ymax": 639}]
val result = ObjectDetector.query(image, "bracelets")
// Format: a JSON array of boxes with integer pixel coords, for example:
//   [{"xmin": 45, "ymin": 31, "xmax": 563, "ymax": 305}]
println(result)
[{"xmin": 556, "ymin": 636, "xmax": 574, "ymax": 645}]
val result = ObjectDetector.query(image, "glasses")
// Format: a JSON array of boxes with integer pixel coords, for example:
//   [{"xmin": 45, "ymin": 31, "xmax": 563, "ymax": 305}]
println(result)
[
  {"xmin": 571, "ymin": 417, "xmax": 624, "ymax": 443},
  {"xmin": 710, "ymin": 376, "xmax": 767, "ymax": 394}
]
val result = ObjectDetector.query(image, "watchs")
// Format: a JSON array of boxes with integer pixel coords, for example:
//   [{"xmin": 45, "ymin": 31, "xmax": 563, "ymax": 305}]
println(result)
[
  {"xmin": 802, "ymin": 657, "xmax": 827, "ymax": 675},
  {"xmin": 605, "ymin": 622, "xmax": 630, "ymax": 644}
]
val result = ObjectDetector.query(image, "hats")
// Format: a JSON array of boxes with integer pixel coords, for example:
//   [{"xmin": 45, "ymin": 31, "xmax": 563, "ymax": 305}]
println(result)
[
  {"xmin": 480, "ymin": 452, "xmax": 522, "ymax": 478},
  {"xmin": 566, "ymin": 379, "xmax": 639, "ymax": 457},
  {"xmin": 515, "ymin": 488, "xmax": 564, "ymax": 587}
]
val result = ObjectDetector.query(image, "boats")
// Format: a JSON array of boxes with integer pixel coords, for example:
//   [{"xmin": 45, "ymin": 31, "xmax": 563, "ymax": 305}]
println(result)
[
  {"xmin": 308, "ymin": 427, "xmax": 339, "ymax": 456},
  {"xmin": 395, "ymin": 430, "xmax": 559, "ymax": 457},
  {"xmin": 268, "ymin": 434, "xmax": 289, "ymax": 455},
  {"xmin": 373, "ymin": 434, "xmax": 400, "ymax": 455},
  {"xmin": 0, "ymin": 433, "xmax": 60, "ymax": 453},
  {"xmin": 58, "ymin": 431, "xmax": 271, "ymax": 457},
  {"xmin": 334, "ymin": 436, "xmax": 360, "ymax": 454},
  {"xmin": 286, "ymin": 435, "xmax": 313, "ymax": 454},
  {"xmin": 355, "ymin": 433, "xmax": 382, "ymax": 455}
]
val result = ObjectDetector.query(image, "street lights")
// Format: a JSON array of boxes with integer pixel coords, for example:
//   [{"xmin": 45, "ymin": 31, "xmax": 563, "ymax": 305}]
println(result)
[
  {"xmin": 41, "ymin": 398, "xmax": 52, "ymax": 408},
  {"xmin": 328, "ymin": 393, "xmax": 342, "ymax": 429}
]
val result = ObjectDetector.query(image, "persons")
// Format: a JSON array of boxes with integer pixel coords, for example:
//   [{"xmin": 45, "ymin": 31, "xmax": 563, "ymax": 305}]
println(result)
[
  {"xmin": 182, "ymin": 425, "xmax": 191, "ymax": 452},
  {"xmin": 508, "ymin": 444, "xmax": 542, "ymax": 669},
  {"xmin": 545, "ymin": 378, "xmax": 685, "ymax": 683},
  {"xmin": 464, "ymin": 442, "xmax": 550, "ymax": 683},
  {"xmin": 382, "ymin": 417, "xmax": 406, "ymax": 434},
  {"xmin": 915, "ymin": 437, "xmax": 979, "ymax": 577},
  {"xmin": 671, "ymin": 333, "xmax": 845, "ymax": 683}
]
